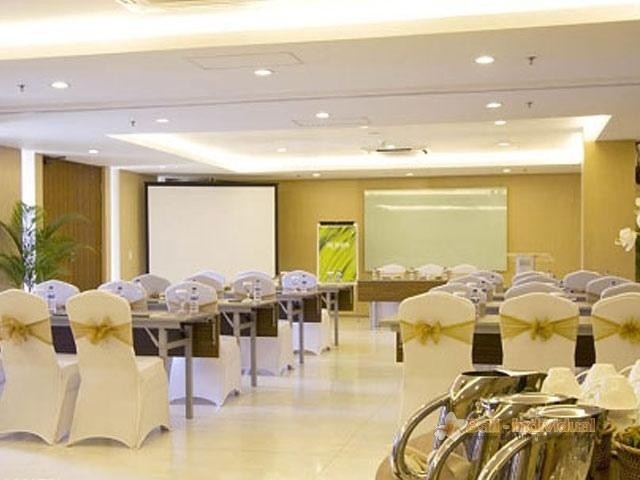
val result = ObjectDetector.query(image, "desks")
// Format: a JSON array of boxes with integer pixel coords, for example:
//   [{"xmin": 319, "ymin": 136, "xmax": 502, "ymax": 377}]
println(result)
[{"xmin": 376, "ymin": 431, "xmax": 454, "ymax": 479}]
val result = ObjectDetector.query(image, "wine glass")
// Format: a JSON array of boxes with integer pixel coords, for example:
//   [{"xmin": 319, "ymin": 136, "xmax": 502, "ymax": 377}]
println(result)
[
  {"xmin": 290, "ymin": 276, "xmax": 300, "ymax": 293},
  {"xmin": 242, "ymin": 281, "xmax": 253, "ymax": 306},
  {"xmin": 175, "ymin": 289, "xmax": 188, "ymax": 317}
]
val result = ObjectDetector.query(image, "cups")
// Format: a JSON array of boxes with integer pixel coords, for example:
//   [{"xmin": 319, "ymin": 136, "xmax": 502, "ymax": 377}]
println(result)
[
  {"xmin": 618, "ymin": 358, "xmax": 640, "ymax": 383},
  {"xmin": 541, "ymin": 367, "xmax": 579, "ymax": 395},
  {"xmin": 576, "ymin": 362, "xmax": 618, "ymax": 386},
  {"xmin": 580, "ymin": 375, "xmax": 635, "ymax": 407}
]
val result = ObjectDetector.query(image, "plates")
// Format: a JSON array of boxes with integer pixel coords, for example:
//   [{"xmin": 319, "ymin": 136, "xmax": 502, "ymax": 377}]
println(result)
[{"xmin": 606, "ymin": 405, "xmax": 639, "ymax": 420}]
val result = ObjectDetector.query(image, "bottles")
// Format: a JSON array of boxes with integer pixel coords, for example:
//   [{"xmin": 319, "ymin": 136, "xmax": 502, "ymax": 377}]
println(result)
[
  {"xmin": 189, "ymin": 286, "xmax": 199, "ymax": 315},
  {"xmin": 469, "ymin": 288, "xmax": 481, "ymax": 319},
  {"xmin": 480, "ymin": 281, "xmax": 488, "ymax": 303},
  {"xmin": 115, "ymin": 285, "xmax": 126, "ymax": 298},
  {"xmin": 570, "ymin": 290, "xmax": 577, "ymax": 305},
  {"xmin": 254, "ymin": 279, "xmax": 262, "ymax": 303},
  {"xmin": 47, "ymin": 286, "xmax": 57, "ymax": 314},
  {"xmin": 135, "ymin": 279, "xmax": 144, "ymax": 290},
  {"xmin": 302, "ymin": 274, "xmax": 308, "ymax": 295}
]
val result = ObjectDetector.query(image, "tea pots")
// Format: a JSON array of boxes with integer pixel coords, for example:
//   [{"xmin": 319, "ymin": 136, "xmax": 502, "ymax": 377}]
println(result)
[
  {"xmin": 390, "ymin": 369, "xmax": 549, "ymax": 480},
  {"xmin": 426, "ymin": 390, "xmax": 578, "ymax": 480},
  {"xmin": 476, "ymin": 403, "xmax": 610, "ymax": 480}
]
